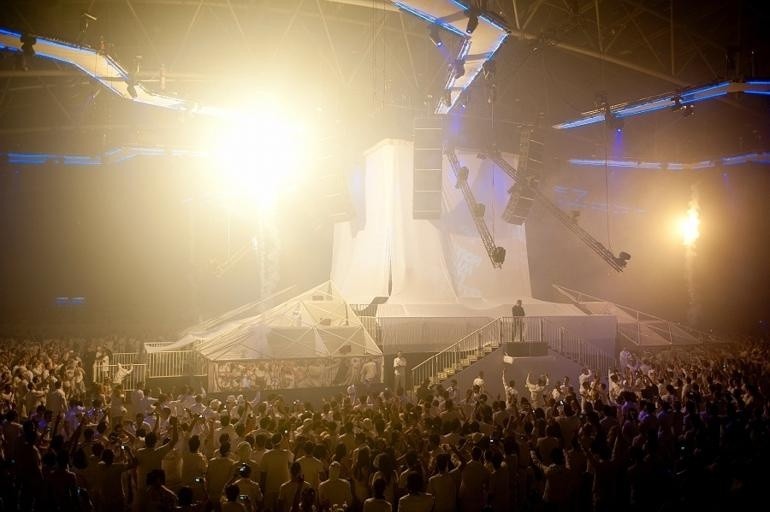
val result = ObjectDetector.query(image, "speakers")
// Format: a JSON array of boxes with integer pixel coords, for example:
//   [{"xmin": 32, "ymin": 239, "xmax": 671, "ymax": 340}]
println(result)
[
  {"xmin": 501, "ymin": 128, "xmax": 544, "ymax": 225},
  {"xmin": 313, "ymin": 128, "xmax": 355, "ymax": 224},
  {"xmin": 412, "ymin": 117, "xmax": 444, "ymax": 220}
]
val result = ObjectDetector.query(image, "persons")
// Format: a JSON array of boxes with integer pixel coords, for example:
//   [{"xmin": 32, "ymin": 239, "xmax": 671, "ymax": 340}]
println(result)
[
  {"xmin": 0, "ymin": 329, "xmax": 770, "ymax": 511},
  {"xmin": 512, "ymin": 300, "xmax": 525, "ymax": 343}
]
